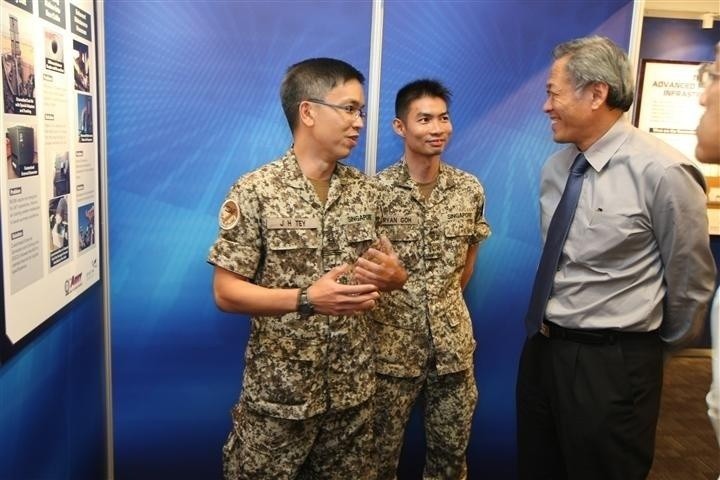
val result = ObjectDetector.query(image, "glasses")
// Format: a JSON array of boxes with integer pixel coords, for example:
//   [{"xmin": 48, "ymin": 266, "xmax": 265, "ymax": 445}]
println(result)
[
  {"xmin": 697, "ymin": 61, "xmax": 720, "ymax": 87},
  {"xmin": 297, "ymin": 99, "xmax": 366, "ymax": 119}
]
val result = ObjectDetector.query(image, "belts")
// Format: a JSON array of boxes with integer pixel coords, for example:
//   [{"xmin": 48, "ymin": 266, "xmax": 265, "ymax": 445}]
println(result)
[{"xmin": 538, "ymin": 319, "xmax": 618, "ymax": 346}]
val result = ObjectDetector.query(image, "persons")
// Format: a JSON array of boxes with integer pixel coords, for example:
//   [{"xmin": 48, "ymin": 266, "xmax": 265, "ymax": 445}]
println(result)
[
  {"xmin": 50, "ymin": 210, "xmax": 68, "ymax": 250},
  {"xmin": 368, "ymin": 78, "xmax": 494, "ymax": 478},
  {"xmin": 57, "ymin": 160, "xmax": 70, "ymax": 192},
  {"xmin": 694, "ymin": 33, "xmax": 720, "ymax": 461},
  {"xmin": 75, "ymin": 45, "xmax": 90, "ymax": 92},
  {"xmin": 204, "ymin": 56, "xmax": 410, "ymax": 478},
  {"xmin": 514, "ymin": 34, "xmax": 717, "ymax": 480}
]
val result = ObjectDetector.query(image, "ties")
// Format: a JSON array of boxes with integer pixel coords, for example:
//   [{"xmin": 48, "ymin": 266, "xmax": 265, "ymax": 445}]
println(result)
[{"xmin": 524, "ymin": 153, "xmax": 591, "ymax": 341}]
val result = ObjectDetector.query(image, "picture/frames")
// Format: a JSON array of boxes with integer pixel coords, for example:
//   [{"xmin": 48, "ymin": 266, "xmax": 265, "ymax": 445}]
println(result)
[
  {"xmin": 633, "ymin": 57, "xmax": 720, "ymax": 243},
  {"xmin": 0, "ymin": 0, "xmax": 104, "ymax": 369}
]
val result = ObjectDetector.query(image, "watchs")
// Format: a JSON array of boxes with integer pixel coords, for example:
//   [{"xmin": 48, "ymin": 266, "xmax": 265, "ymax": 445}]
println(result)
[{"xmin": 295, "ymin": 285, "xmax": 316, "ymax": 320}]
